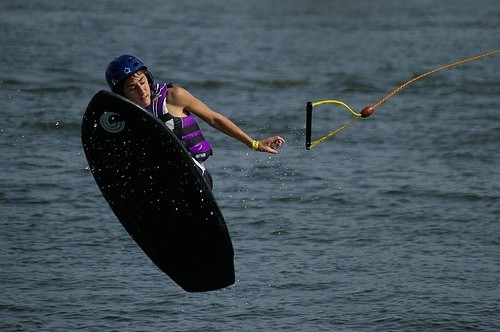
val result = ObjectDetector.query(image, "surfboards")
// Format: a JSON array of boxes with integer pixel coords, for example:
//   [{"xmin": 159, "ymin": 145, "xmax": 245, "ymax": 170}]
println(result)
[{"xmin": 80, "ymin": 89, "xmax": 236, "ymax": 292}]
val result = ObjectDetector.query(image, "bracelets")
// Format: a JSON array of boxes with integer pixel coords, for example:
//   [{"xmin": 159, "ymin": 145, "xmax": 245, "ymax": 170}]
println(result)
[{"xmin": 252, "ymin": 139, "xmax": 259, "ymax": 151}]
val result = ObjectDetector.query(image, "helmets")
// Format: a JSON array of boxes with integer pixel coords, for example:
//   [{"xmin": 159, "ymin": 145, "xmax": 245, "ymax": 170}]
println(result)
[{"xmin": 105, "ymin": 54, "xmax": 148, "ymax": 92}]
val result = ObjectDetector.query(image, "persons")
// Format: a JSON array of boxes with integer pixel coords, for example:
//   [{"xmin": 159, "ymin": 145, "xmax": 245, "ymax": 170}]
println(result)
[{"xmin": 105, "ymin": 54, "xmax": 285, "ymax": 190}]
